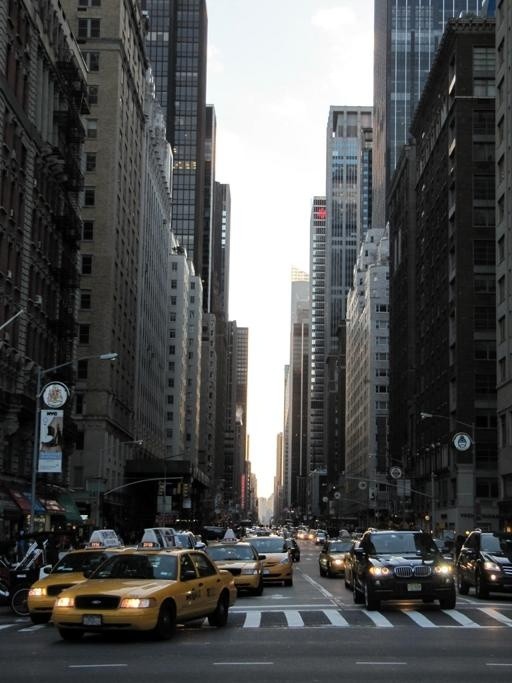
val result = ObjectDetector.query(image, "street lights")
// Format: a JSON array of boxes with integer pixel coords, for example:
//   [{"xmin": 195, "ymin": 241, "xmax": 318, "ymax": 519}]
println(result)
[
  {"xmin": 362, "ymin": 450, "xmax": 409, "ymax": 522},
  {"xmin": 26, "ymin": 347, "xmax": 120, "ymax": 534},
  {"xmin": 91, "ymin": 435, "xmax": 144, "ymax": 528},
  {"xmin": 417, "ymin": 408, "xmax": 479, "ymax": 510}
]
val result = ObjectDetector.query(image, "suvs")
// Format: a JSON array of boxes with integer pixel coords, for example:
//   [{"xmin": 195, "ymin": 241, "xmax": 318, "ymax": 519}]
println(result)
[{"xmin": 354, "ymin": 525, "xmax": 450, "ymax": 610}]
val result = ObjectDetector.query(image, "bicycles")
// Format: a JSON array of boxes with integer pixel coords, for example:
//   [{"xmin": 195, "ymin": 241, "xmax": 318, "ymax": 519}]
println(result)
[{"xmin": 0, "ymin": 586, "xmax": 35, "ymax": 619}]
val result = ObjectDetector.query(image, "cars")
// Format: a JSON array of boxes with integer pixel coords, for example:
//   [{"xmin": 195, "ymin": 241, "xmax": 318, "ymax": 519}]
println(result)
[
  {"xmin": 57, "ymin": 545, "xmax": 238, "ymax": 642},
  {"xmin": 457, "ymin": 528, "xmax": 512, "ymax": 598},
  {"xmin": 318, "ymin": 534, "xmax": 360, "ymax": 576},
  {"xmin": 252, "ymin": 521, "xmax": 326, "ymax": 543},
  {"xmin": 248, "ymin": 541, "xmax": 293, "ymax": 581},
  {"xmin": 205, "ymin": 537, "xmax": 261, "ymax": 595},
  {"xmin": 31, "ymin": 547, "xmax": 114, "ymax": 620}
]
